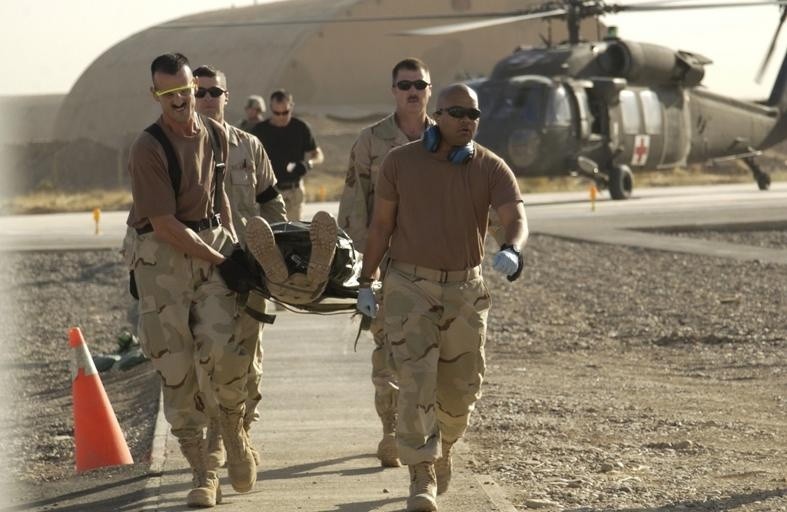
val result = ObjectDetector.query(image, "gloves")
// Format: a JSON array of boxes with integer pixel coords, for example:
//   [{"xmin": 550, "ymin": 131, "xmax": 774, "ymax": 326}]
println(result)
[
  {"xmin": 492, "ymin": 247, "xmax": 519, "ymax": 277},
  {"xmin": 356, "ymin": 287, "xmax": 378, "ymax": 320},
  {"xmin": 215, "ymin": 247, "xmax": 264, "ymax": 294}
]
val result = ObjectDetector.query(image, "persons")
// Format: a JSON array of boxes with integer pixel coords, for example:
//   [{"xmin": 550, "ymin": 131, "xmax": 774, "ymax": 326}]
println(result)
[
  {"xmin": 251, "ymin": 89, "xmax": 325, "ymax": 221},
  {"xmin": 356, "ymin": 84, "xmax": 528, "ymax": 511},
  {"xmin": 244, "ymin": 211, "xmax": 355, "ymax": 304},
  {"xmin": 336, "ymin": 57, "xmax": 437, "ymax": 469},
  {"xmin": 124, "ymin": 52, "xmax": 286, "ymax": 506}
]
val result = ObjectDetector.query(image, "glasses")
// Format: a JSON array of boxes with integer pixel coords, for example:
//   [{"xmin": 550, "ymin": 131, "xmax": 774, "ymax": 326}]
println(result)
[
  {"xmin": 446, "ymin": 105, "xmax": 481, "ymax": 120},
  {"xmin": 269, "ymin": 104, "xmax": 290, "ymax": 115},
  {"xmin": 152, "ymin": 81, "xmax": 194, "ymax": 99},
  {"xmin": 394, "ymin": 79, "xmax": 430, "ymax": 91},
  {"xmin": 194, "ymin": 87, "xmax": 226, "ymax": 98}
]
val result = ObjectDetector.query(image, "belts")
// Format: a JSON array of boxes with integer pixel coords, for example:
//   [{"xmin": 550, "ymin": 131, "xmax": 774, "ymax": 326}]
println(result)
[
  {"xmin": 391, "ymin": 260, "xmax": 482, "ymax": 283},
  {"xmin": 279, "ymin": 182, "xmax": 301, "ymax": 190},
  {"xmin": 135, "ymin": 217, "xmax": 220, "ymax": 234}
]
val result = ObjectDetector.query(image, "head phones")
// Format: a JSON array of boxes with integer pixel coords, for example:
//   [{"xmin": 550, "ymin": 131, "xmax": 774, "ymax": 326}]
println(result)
[{"xmin": 423, "ymin": 124, "xmax": 475, "ymax": 164}]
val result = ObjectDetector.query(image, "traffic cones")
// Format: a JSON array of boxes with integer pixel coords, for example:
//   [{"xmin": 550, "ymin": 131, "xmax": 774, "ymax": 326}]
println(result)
[{"xmin": 64, "ymin": 324, "xmax": 135, "ymax": 471}]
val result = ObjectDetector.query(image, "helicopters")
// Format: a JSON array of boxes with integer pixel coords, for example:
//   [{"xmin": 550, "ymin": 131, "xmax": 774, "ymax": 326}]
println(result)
[{"xmin": 150, "ymin": 0, "xmax": 785, "ymax": 204}]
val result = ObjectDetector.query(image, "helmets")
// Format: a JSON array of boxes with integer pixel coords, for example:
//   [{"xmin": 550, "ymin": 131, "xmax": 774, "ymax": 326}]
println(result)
[{"xmin": 244, "ymin": 95, "xmax": 266, "ymax": 113}]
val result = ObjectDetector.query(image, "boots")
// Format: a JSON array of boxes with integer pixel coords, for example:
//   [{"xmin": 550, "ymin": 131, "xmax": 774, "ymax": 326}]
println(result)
[
  {"xmin": 434, "ymin": 438, "xmax": 457, "ymax": 493},
  {"xmin": 171, "ymin": 392, "xmax": 261, "ymax": 506},
  {"xmin": 379, "ymin": 413, "xmax": 403, "ymax": 467},
  {"xmin": 407, "ymin": 461, "xmax": 437, "ymax": 511}
]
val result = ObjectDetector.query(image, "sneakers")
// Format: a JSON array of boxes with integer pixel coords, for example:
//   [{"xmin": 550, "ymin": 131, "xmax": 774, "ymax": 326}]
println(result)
[
  {"xmin": 307, "ymin": 210, "xmax": 338, "ymax": 285},
  {"xmin": 245, "ymin": 215, "xmax": 288, "ymax": 283}
]
